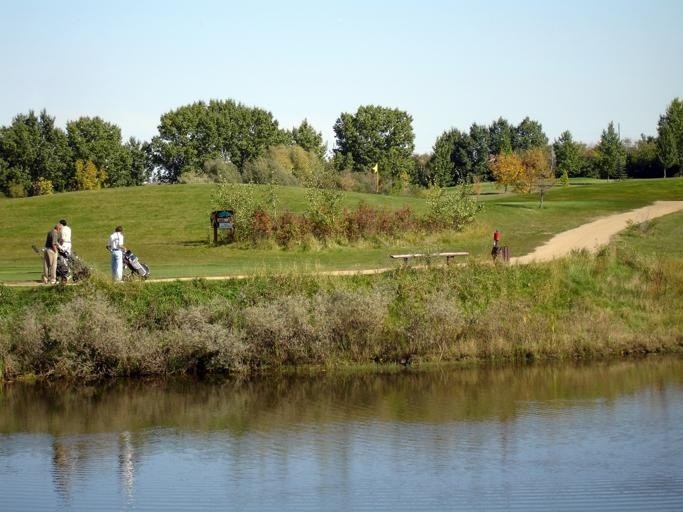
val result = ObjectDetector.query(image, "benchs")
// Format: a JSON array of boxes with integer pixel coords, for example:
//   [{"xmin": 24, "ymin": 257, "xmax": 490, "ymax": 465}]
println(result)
[{"xmin": 390, "ymin": 252, "xmax": 469, "ymax": 265}]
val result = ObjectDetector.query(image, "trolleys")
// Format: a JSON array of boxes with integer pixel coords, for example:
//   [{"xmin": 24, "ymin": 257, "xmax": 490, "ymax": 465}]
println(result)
[
  {"xmin": 106, "ymin": 245, "xmax": 152, "ymax": 281},
  {"xmin": 31, "ymin": 244, "xmax": 72, "ymax": 284}
]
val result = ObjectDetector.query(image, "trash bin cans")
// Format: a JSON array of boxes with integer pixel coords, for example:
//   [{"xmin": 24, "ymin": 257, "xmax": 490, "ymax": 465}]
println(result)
[{"xmin": 493, "ymin": 246, "xmax": 509, "ymax": 263}]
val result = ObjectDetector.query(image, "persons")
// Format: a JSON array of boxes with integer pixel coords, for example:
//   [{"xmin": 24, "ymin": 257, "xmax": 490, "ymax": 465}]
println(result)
[
  {"xmin": 56, "ymin": 219, "xmax": 72, "ymax": 281},
  {"xmin": 39, "ymin": 224, "xmax": 61, "ymax": 285},
  {"xmin": 105, "ymin": 225, "xmax": 125, "ymax": 283}
]
